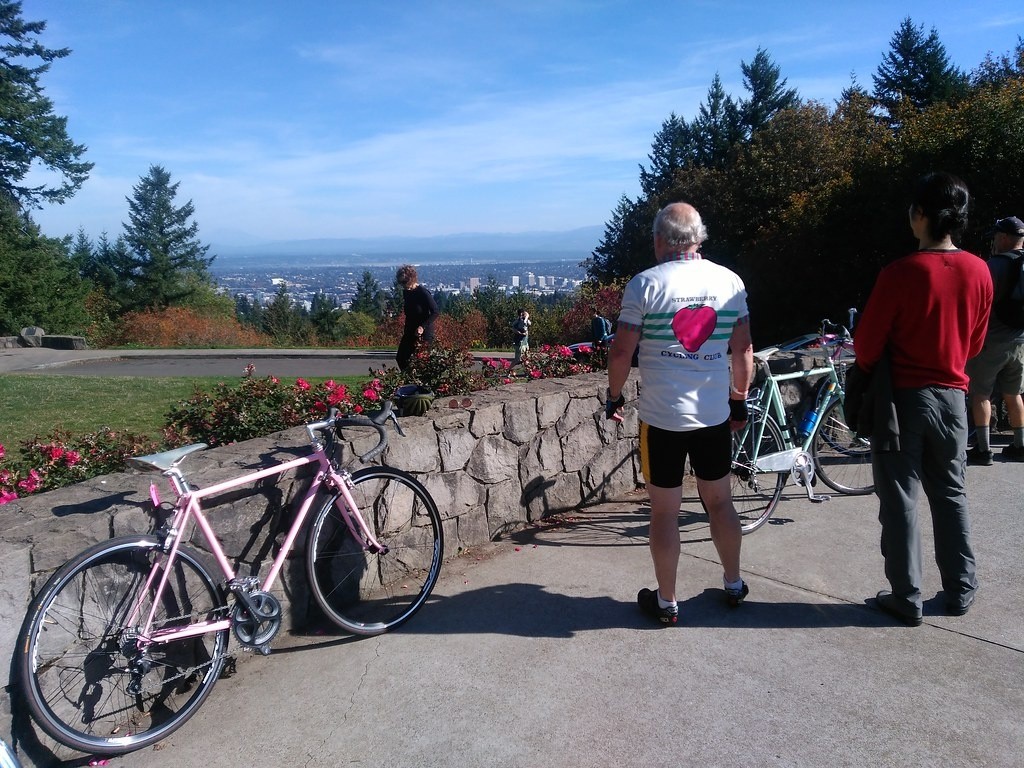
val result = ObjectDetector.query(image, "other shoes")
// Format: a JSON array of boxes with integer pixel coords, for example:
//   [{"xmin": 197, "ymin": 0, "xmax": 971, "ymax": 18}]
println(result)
[
  {"xmin": 877, "ymin": 590, "xmax": 923, "ymax": 626},
  {"xmin": 944, "ymin": 591, "xmax": 976, "ymax": 616},
  {"xmin": 965, "ymin": 444, "xmax": 994, "ymax": 466},
  {"xmin": 1001, "ymin": 442, "xmax": 1024, "ymax": 462}
]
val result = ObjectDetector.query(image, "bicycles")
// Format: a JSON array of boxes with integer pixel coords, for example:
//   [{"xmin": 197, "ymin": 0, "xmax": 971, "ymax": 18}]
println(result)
[
  {"xmin": 11, "ymin": 396, "xmax": 444, "ymax": 759},
  {"xmin": 695, "ymin": 307, "xmax": 978, "ymax": 536}
]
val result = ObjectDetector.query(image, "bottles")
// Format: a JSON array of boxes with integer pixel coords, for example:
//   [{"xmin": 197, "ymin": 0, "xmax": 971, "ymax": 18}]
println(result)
[{"xmin": 797, "ymin": 407, "xmax": 819, "ymax": 437}]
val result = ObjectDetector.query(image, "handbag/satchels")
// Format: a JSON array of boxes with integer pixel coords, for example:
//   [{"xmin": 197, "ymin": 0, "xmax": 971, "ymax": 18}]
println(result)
[{"xmin": 994, "ymin": 296, "xmax": 1024, "ymax": 332}]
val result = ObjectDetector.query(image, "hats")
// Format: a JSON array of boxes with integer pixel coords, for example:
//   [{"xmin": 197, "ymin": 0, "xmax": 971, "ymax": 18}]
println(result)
[{"xmin": 992, "ymin": 215, "xmax": 1024, "ymax": 237}]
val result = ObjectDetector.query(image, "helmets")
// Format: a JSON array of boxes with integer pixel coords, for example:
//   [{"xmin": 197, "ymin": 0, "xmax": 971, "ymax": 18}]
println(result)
[{"xmin": 394, "ymin": 383, "xmax": 436, "ymax": 417}]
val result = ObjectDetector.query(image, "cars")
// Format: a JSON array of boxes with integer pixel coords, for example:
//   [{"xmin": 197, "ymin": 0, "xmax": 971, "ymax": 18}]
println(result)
[{"xmin": 566, "ymin": 333, "xmax": 615, "ymax": 368}]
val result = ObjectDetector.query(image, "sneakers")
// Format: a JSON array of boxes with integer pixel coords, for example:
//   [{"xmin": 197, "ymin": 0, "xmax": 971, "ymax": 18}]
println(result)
[
  {"xmin": 637, "ymin": 588, "xmax": 679, "ymax": 627},
  {"xmin": 726, "ymin": 580, "xmax": 749, "ymax": 607}
]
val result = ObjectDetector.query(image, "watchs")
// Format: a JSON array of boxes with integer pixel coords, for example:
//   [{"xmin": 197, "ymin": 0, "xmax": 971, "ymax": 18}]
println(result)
[{"xmin": 606, "ymin": 387, "xmax": 621, "ymax": 402}]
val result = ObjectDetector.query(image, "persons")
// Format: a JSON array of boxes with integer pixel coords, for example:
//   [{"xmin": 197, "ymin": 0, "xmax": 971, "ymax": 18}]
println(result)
[
  {"xmin": 507, "ymin": 308, "xmax": 532, "ymax": 372},
  {"xmin": 605, "ymin": 203, "xmax": 755, "ymax": 624},
  {"xmin": 394, "ymin": 264, "xmax": 439, "ymax": 375},
  {"xmin": 965, "ymin": 216, "xmax": 1024, "ymax": 467},
  {"xmin": 850, "ymin": 171, "xmax": 995, "ymax": 626},
  {"xmin": 590, "ymin": 308, "xmax": 608, "ymax": 372}
]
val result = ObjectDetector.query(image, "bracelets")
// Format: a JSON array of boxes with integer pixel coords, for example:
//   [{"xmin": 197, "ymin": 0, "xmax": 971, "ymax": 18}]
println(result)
[{"xmin": 731, "ymin": 386, "xmax": 748, "ymax": 400}]
